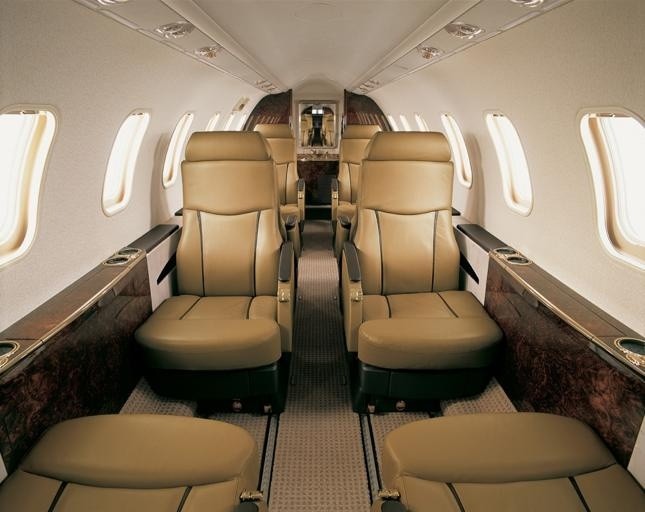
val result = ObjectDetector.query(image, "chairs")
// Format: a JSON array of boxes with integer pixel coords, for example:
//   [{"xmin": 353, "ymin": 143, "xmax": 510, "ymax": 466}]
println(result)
[
  {"xmin": 329, "ymin": 124, "xmax": 391, "ymax": 257},
  {"xmin": 1, "ymin": 411, "xmax": 268, "ymax": 511},
  {"xmin": 136, "ymin": 128, "xmax": 301, "ymax": 412},
  {"xmin": 251, "ymin": 123, "xmax": 307, "ymax": 258},
  {"xmin": 370, "ymin": 410, "xmax": 643, "ymax": 511},
  {"xmin": 339, "ymin": 129, "xmax": 508, "ymax": 412}
]
